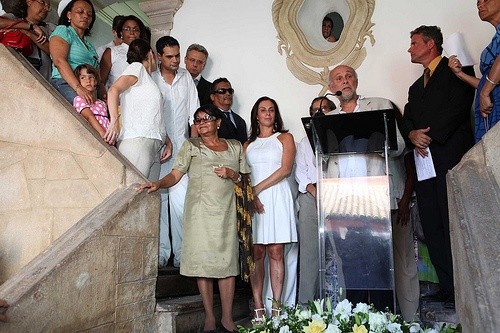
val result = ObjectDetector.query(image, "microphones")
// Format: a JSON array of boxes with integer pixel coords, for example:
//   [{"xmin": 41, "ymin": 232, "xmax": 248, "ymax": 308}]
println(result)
[{"xmin": 312, "ymin": 91, "xmax": 342, "ymax": 116}]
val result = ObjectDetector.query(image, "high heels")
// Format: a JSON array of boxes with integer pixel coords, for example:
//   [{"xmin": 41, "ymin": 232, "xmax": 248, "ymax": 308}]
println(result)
[
  {"xmin": 271, "ymin": 307, "xmax": 283, "ymax": 320},
  {"xmin": 251, "ymin": 305, "xmax": 266, "ymax": 326}
]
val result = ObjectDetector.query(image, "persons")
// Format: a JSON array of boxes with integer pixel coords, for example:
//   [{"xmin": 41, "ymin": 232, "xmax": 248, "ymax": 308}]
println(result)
[
  {"xmin": 322, "ymin": 17, "xmax": 336, "ymax": 42},
  {"xmin": 403, "ymin": 25, "xmax": 475, "ymax": 308},
  {"xmin": 184, "ymin": 44, "xmax": 247, "ymax": 146},
  {"xmin": 73, "ymin": 63, "xmax": 115, "ymax": 145},
  {"xmin": 96, "ymin": 15, "xmax": 151, "ymax": 131},
  {"xmin": 312, "ymin": 65, "xmax": 406, "ymax": 314},
  {"xmin": 448, "ymin": 0, "xmax": 500, "ymax": 144},
  {"xmin": 49, "ymin": 0, "xmax": 106, "ymax": 107},
  {"xmin": 104, "ymin": 39, "xmax": 173, "ymax": 183},
  {"xmin": 295, "ymin": 96, "xmax": 346, "ymax": 305},
  {"xmin": 0, "ymin": 0, "xmax": 72, "ymax": 80},
  {"xmin": 243, "ymin": 96, "xmax": 300, "ymax": 325},
  {"xmin": 141, "ymin": 106, "xmax": 251, "ymax": 333},
  {"xmin": 151, "ymin": 36, "xmax": 200, "ymax": 268}
]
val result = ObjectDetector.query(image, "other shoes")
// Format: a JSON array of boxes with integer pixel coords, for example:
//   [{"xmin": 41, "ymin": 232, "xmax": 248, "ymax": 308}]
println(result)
[
  {"xmin": 174, "ymin": 260, "xmax": 180, "ymax": 267},
  {"xmin": 158, "ymin": 256, "xmax": 165, "ymax": 268},
  {"xmin": 220, "ymin": 320, "xmax": 239, "ymax": 333},
  {"xmin": 202, "ymin": 324, "xmax": 218, "ymax": 333}
]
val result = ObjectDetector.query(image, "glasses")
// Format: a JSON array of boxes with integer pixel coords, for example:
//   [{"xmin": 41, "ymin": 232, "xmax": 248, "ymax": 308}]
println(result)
[
  {"xmin": 311, "ymin": 106, "xmax": 330, "ymax": 115},
  {"xmin": 212, "ymin": 88, "xmax": 234, "ymax": 95},
  {"xmin": 193, "ymin": 115, "xmax": 217, "ymax": 125},
  {"xmin": 122, "ymin": 27, "xmax": 141, "ymax": 33},
  {"xmin": 187, "ymin": 57, "xmax": 205, "ymax": 66},
  {"xmin": 33, "ymin": 0, "xmax": 52, "ymax": 12}
]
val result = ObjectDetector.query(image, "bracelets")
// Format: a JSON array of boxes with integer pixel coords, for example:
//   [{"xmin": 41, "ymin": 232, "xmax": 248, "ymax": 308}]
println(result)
[
  {"xmin": 75, "ymin": 85, "xmax": 82, "ymax": 91},
  {"xmin": 29, "ymin": 24, "xmax": 34, "ymax": 30},
  {"xmin": 486, "ymin": 74, "xmax": 500, "ymax": 84},
  {"xmin": 234, "ymin": 173, "xmax": 242, "ymax": 184}
]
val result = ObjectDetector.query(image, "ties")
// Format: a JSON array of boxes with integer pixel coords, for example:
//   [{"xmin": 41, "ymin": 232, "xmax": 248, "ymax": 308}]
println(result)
[
  {"xmin": 225, "ymin": 112, "xmax": 230, "ymax": 121},
  {"xmin": 424, "ymin": 67, "xmax": 430, "ymax": 86}
]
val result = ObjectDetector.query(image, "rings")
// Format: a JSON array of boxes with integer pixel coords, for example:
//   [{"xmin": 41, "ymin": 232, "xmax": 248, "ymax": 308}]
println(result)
[
  {"xmin": 221, "ymin": 175, "xmax": 223, "ymax": 178},
  {"xmin": 455, "ymin": 61, "xmax": 457, "ymax": 63}
]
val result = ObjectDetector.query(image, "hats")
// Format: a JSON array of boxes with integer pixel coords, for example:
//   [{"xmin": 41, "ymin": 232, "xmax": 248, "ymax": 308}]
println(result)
[{"xmin": 58, "ymin": 0, "xmax": 72, "ymax": 17}]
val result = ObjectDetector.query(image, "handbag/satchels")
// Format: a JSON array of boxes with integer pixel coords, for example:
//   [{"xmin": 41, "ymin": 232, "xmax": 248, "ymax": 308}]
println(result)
[{"xmin": 0, "ymin": 19, "xmax": 33, "ymax": 56}]
